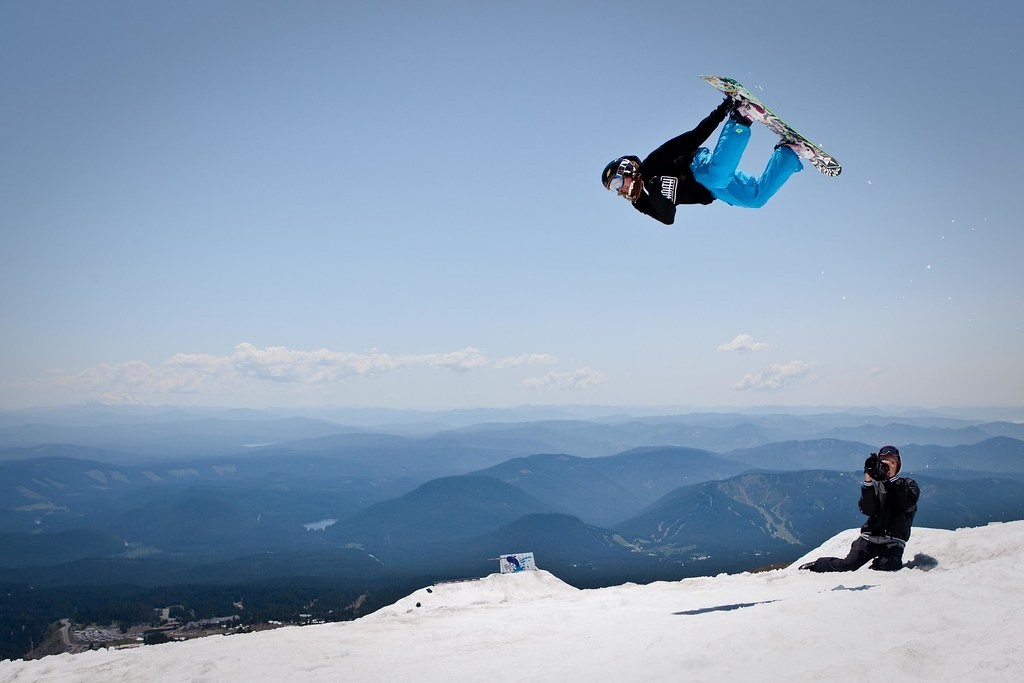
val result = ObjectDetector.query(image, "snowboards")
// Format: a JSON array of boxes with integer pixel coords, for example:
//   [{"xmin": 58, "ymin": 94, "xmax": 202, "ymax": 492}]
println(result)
[{"xmin": 697, "ymin": 73, "xmax": 843, "ymax": 178}]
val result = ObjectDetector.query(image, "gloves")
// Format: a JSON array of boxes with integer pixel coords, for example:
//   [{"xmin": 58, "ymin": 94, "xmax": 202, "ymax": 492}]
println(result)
[
  {"xmin": 717, "ymin": 96, "xmax": 732, "ymax": 115},
  {"xmin": 648, "ymin": 176, "xmax": 662, "ymax": 195}
]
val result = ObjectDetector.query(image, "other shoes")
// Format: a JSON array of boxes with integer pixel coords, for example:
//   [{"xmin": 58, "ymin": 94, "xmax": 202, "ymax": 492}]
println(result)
[{"xmin": 799, "ymin": 558, "xmax": 823, "ymax": 571}]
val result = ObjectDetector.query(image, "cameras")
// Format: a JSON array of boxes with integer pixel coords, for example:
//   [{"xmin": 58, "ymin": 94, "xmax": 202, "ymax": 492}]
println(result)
[{"xmin": 863, "ymin": 452, "xmax": 890, "ymax": 482}]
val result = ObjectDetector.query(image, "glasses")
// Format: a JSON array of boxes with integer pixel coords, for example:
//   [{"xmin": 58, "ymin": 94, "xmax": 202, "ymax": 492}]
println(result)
[
  {"xmin": 609, "ymin": 174, "xmax": 624, "ymax": 194},
  {"xmin": 879, "ymin": 446, "xmax": 899, "ymax": 457}
]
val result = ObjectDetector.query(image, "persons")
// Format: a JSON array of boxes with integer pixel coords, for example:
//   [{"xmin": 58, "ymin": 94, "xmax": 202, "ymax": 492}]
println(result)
[
  {"xmin": 799, "ymin": 445, "xmax": 920, "ymax": 572},
  {"xmin": 601, "ymin": 96, "xmax": 816, "ymax": 226}
]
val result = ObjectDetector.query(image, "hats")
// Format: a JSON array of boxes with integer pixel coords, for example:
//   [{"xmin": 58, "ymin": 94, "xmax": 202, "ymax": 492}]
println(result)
[
  {"xmin": 877, "ymin": 455, "xmax": 902, "ymax": 475},
  {"xmin": 601, "ymin": 155, "xmax": 641, "ymax": 190}
]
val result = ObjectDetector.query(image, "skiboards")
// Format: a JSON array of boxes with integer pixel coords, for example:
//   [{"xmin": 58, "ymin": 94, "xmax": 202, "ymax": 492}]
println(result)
[{"xmin": 673, "ymin": 582, "xmax": 881, "ymax": 616}]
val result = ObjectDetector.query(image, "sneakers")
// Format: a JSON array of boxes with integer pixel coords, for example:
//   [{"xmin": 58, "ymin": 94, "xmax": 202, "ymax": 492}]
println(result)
[
  {"xmin": 731, "ymin": 102, "xmax": 764, "ymax": 123},
  {"xmin": 775, "ymin": 138, "xmax": 815, "ymax": 160}
]
